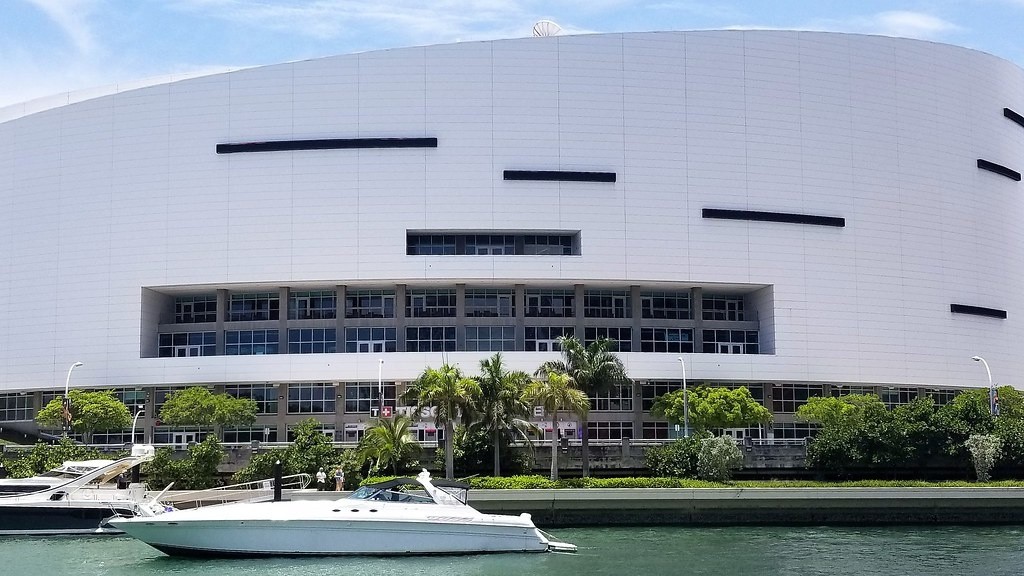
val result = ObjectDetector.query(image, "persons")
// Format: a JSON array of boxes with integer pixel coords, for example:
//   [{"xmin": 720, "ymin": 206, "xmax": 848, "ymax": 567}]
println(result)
[
  {"xmin": 992, "ymin": 388, "xmax": 1000, "ymax": 415},
  {"xmin": 334, "ymin": 466, "xmax": 345, "ymax": 490},
  {"xmin": 315, "ymin": 467, "xmax": 327, "ymax": 491},
  {"xmin": 117, "ymin": 470, "xmax": 128, "ymax": 489}
]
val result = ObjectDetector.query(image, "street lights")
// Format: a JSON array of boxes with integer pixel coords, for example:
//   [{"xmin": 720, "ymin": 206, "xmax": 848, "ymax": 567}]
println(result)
[
  {"xmin": 378, "ymin": 360, "xmax": 385, "ymax": 423},
  {"xmin": 64, "ymin": 361, "xmax": 83, "ymax": 441},
  {"xmin": 677, "ymin": 357, "xmax": 689, "ymax": 439},
  {"xmin": 972, "ymin": 356, "xmax": 993, "ymax": 415},
  {"xmin": 131, "ymin": 410, "xmax": 145, "ymax": 445}
]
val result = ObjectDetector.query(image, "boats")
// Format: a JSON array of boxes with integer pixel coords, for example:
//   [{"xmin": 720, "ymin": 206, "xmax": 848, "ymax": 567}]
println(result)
[
  {"xmin": 107, "ymin": 497, "xmax": 579, "ymax": 563},
  {"xmin": 0, "ymin": 458, "xmax": 311, "ymax": 537}
]
